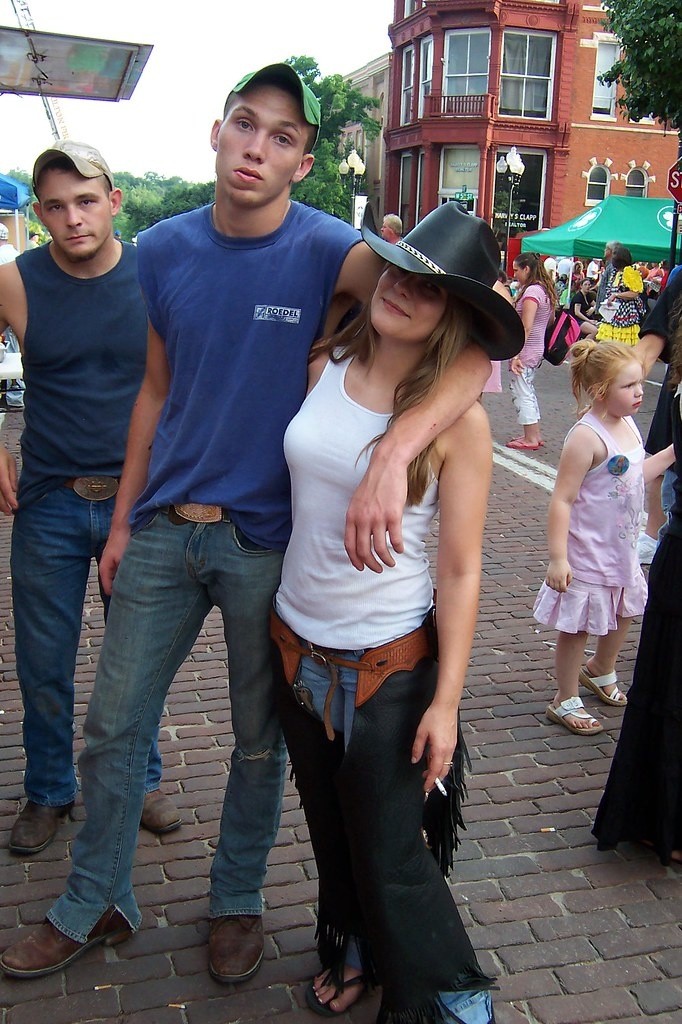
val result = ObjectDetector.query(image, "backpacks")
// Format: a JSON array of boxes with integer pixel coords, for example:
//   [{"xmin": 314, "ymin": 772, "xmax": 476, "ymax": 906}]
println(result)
[{"xmin": 531, "ymin": 282, "xmax": 581, "ymax": 366}]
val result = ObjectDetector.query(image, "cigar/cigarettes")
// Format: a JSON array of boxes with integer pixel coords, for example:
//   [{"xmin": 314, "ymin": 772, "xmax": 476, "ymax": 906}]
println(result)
[{"xmin": 435, "ymin": 777, "xmax": 447, "ymax": 797}]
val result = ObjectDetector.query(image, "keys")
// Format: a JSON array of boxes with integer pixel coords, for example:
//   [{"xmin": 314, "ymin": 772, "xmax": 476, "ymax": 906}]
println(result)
[{"xmin": 296, "ymin": 688, "xmax": 313, "ymax": 712}]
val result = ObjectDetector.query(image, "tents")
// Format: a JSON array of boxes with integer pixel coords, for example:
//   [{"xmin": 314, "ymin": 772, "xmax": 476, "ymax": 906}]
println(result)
[
  {"xmin": 521, "ymin": 195, "xmax": 682, "ymax": 304},
  {"xmin": 0, "ymin": 173, "xmax": 30, "ymax": 251}
]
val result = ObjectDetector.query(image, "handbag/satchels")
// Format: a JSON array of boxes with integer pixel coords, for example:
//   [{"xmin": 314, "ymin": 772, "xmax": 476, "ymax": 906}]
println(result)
[{"xmin": 591, "ymin": 311, "xmax": 603, "ymax": 322}]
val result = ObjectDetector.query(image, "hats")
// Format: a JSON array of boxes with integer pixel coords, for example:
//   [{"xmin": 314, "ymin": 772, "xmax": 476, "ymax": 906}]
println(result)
[
  {"xmin": 31, "ymin": 232, "xmax": 39, "ymax": 238},
  {"xmin": 224, "ymin": 63, "xmax": 321, "ymax": 156},
  {"xmin": 362, "ymin": 201, "xmax": 527, "ymax": 360},
  {"xmin": 131, "ymin": 236, "xmax": 137, "ymax": 242},
  {"xmin": 32, "ymin": 140, "xmax": 114, "ymax": 199},
  {"xmin": 114, "ymin": 229, "xmax": 121, "ymax": 236},
  {"xmin": 0, "ymin": 221, "xmax": 9, "ymax": 239}
]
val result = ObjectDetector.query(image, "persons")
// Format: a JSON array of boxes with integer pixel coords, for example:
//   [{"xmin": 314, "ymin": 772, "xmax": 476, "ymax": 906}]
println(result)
[
  {"xmin": 543, "ymin": 241, "xmax": 669, "ymax": 346},
  {"xmin": 505, "ymin": 252, "xmax": 559, "ymax": 450},
  {"xmin": 0, "ymin": 223, "xmax": 20, "ymax": 264},
  {"xmin": 0, "ymin": 141, "xmax": 183, "ymax": 853},
  {"xmin": 578, "ymin": 269, "xmax": 682, "ymax": 565},
  {"xmin": 115, "ymin": 231, "xmax": 125, "ymax": 241},
  {"xmin": 0, "ymin": 64, "xmax": 493, "ymax": 983},
  {"xmin": 380, "ymin": 213, "xmax": 403, "ymax": 244},
  {"xmin": 267, "ymin": 200, "xmax": 525, "ymax": 1024},
  {"xmin": 29, "ymin": 233, "xmax": 40, "ymax": 249},
  {"xmin": 476, "ymin": 270, "xmax": 521, "ymax": 403},
  {"xmin": 534, "ymin": 338, "xmax": 676, "ymax": 735},
  {"xmin": 589, "ymin": 316, "xmax": 682, "ymax": 867}
]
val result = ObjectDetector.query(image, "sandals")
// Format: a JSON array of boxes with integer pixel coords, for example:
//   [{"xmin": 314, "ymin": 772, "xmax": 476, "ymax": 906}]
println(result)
[{"xmin": 306, "ymin": 963, "xmax": 372, "ymax": 1019}]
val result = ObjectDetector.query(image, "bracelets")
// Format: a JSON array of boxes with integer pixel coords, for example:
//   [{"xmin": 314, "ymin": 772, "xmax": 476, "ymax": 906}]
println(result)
[{"xmin": 511, "ymin": 357, "xmax": 520, "ymax": 361}]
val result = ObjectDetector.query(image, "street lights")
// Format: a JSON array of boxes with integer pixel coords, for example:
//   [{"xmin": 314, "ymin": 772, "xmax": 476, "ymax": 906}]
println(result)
[
  {"xmin": 496, "ymin": 146, "xmax": 525, "ymax": 274},
  {"xmin": 338, "ymin": 150, "xmax": 366, "ymax": 227}
]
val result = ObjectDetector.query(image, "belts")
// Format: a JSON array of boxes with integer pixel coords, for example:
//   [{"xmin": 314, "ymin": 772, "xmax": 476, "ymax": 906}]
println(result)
[
  {"xmin": 157, "ymin": 503, "xmax": 230, "ymax": 526},
  {"xmin": 63, "ymin": 476, "xmax": 121, "ymax": 501}
]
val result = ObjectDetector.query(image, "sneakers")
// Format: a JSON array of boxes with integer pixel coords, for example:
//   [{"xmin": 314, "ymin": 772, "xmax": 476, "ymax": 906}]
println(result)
[{"xmin": 636, "ymin": 533, "xmax": 658, "ymax": 565}]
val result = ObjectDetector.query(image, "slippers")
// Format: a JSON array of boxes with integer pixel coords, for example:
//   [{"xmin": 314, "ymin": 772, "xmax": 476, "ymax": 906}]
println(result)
[
  {"xmin": 506, "ymin": 438, "xmax": 539, "ymax": 450},
  {"xmin": 511, "ymin": 435, "xmax": 544, "ymax": 446},
  {"xmin": 546, "ymin": 695, "xmax": 603, "ymax": 735},
  {"xmin": 579, "ymin": 666, "xmax": 627, "ymax": 706}
]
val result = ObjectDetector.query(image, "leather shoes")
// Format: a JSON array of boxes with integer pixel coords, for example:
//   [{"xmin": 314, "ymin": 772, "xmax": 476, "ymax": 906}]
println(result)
[
  {"xmin": 9, "ymin": 798, "xmax": 76, "ymax": 852},
  {"xmin": 0, "ymin": 901, "xmax": 131, "ymax": 978},
  {"xmin": 207, "ymin": 910, "xmax": 264, "ymax": 981},
  {"xmin": 140, "ymin": 790, "xmax": 182, "ymax": 833}
]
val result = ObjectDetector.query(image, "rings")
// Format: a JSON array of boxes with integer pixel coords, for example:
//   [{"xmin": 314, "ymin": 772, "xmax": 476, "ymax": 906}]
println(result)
[{"xmin": 444, "ymin": 763, "xmax": 450, "ymax": 765}]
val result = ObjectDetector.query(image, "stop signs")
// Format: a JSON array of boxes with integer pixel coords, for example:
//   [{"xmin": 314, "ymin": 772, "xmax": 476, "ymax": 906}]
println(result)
[{"xmin": 667, "ymin": 157, "xmax": 682, "ymax": 203}]
